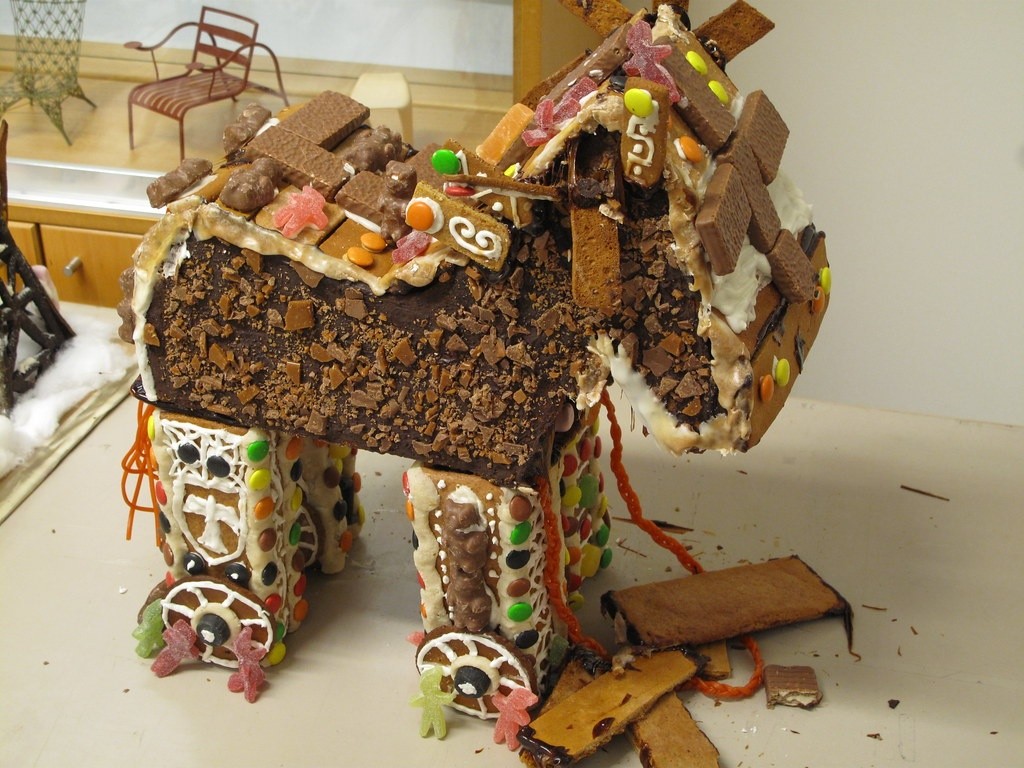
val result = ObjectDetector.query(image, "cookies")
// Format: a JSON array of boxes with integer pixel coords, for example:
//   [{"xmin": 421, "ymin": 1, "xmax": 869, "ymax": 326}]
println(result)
[{"xmin": 514, "ymin": 555, "xmax": 850, "ymax": 768}]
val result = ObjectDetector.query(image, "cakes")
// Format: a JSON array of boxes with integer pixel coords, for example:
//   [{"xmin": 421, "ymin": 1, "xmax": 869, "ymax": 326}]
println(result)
[{"xmin": 116, "ymin": 0, "xmax": 830, "ymax": 743}]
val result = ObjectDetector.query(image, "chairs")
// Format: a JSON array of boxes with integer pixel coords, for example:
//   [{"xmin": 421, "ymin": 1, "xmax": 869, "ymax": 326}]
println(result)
[{"xmin": 124, "ymin": 7, "xmax": 294, "ymax": 166}]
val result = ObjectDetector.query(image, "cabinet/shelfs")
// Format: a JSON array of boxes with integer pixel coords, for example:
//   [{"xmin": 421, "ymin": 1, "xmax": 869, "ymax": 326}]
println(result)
[{"xmin": 0, "ymin": 201, "xmax": 163, "ymax": 310}]
